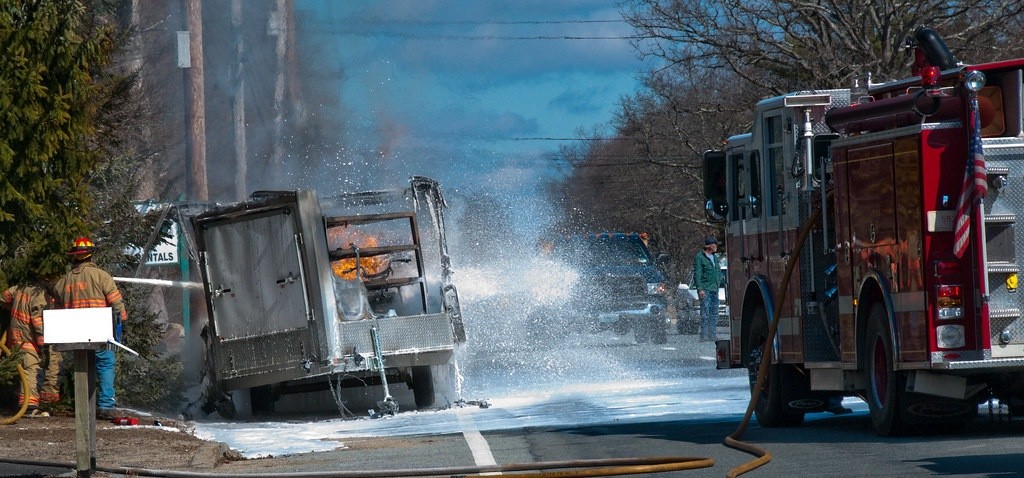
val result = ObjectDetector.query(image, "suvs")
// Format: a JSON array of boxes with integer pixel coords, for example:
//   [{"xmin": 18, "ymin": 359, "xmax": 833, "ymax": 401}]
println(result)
[{"xmin": 527, "ymin": 234, "xmax": 668, "ymax": 346}]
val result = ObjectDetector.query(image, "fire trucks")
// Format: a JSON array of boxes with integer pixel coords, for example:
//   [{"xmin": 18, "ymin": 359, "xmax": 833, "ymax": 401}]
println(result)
[{"xmin": 699, "ymin": 25, "xmax": 1023, "ymax": 434}]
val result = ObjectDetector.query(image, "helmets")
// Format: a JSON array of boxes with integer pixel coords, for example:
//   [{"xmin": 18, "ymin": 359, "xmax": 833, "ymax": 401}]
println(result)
[{"xmin": 64, "ymin": 237, "xmax": 96, "ymax": 254}]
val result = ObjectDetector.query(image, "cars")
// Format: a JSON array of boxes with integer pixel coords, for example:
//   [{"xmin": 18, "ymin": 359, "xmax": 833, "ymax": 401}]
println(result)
[{"xmin": 677, "ymin": 266, "xmax": 733, "ymax": 336}]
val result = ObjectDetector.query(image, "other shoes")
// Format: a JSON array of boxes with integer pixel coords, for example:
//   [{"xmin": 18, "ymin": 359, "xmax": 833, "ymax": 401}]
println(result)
[
  {"xmin": 39, "ymin": 402, "xmax": 69, "ymax": 417},
  {"xmin": 96, "ymin": 409, "xmax": 128, "ymax": 420},
  {"xmin": 17, "ymin": 407, "xmax": 50, "ymax": 417},
  {"xmin": 708, "ymin": 334, "xmax": 718, "ymax": 341},
  {"xmin": 699, "ymin": 335, "xmax": 711, "ymax": 342}
]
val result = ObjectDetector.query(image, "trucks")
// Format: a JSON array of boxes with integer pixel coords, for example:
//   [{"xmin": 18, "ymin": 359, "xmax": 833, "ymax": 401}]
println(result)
[{"xmin": 171, "ymin": 178, "xmax": 468, "ymax": 419}]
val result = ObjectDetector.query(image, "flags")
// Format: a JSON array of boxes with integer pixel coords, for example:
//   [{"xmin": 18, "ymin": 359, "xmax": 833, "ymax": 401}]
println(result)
[{"xmin": 951, "ymin": 101, "xmax": 986, "ymax": 259}]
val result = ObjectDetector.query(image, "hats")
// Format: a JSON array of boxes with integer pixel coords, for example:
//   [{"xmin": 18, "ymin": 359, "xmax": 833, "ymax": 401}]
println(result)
[{"xmin": 705, "ymin": 237, "xmax": 722, "ymax": 245}]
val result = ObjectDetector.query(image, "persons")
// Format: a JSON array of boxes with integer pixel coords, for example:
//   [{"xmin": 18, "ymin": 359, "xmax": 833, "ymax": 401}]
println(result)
[
  {"xmin": 694, "ymin": 237, "xmax": 722, "ymax": 342},
  {"xmin": 0, "ymin": 236, "xmax": 128, "ymax": 419}
]
